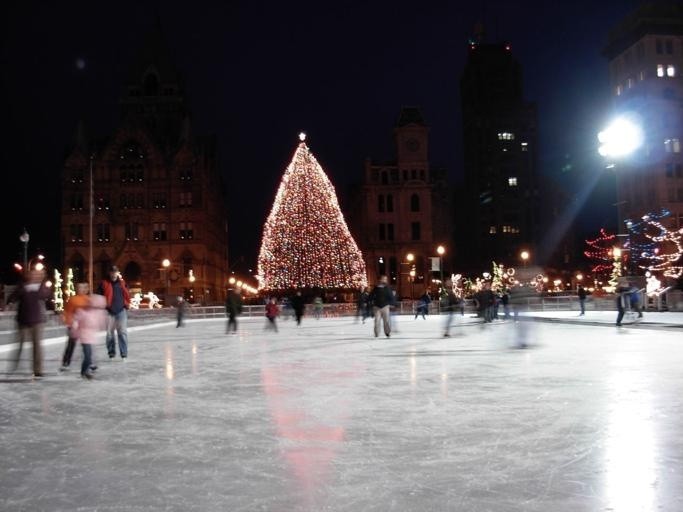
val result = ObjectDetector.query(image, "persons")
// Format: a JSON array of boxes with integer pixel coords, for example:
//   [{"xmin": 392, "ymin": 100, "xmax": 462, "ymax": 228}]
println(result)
[
  {"xmin": 97, "ymin": 265, "xmax": 131, "ymax": 358},
  {"xmin": 358, "ymin": 274, "xmax": 397, "ymax": 338},
  {"xmin": 176, "ymin": 296, "xmax": 190, "ymax": 328},
  {"xmin": 461, "ymin": 295, "xmax": 466, "ymax": 316},
  {"xmin": 225, "ymin": 289, "xmax": 243, "ymax": 331},
  {"xmin": 472, "ymin": 282, "xmax": 511, "ymax": 322},
  {"xmin": 59, "ymin": 279, "xmax": 107, "ymax": 377},
  {"xmin": 414, "ymin": 290, "xmax": 432, "ymax": 320},
  {"xmin": 264, "ymin": 295, "xmax": 280, "ymax": 332},
  {"xmin": 312, "ymin": 296, "xmax": 323, "ymax": 320},
  {"xmin": 577, "ymin": 285, "xmax": 586, "ymax": 314},
  {"xmin": 616, "ymin": 288, "xmax": 643, "ymax": 327},
  {"xmin": 290, "ymin": 291, "xmax": 305, "ymax": 325},
  {"xmin": 5, "ymin": 270, "xmax": 51, "ymax": 378}
]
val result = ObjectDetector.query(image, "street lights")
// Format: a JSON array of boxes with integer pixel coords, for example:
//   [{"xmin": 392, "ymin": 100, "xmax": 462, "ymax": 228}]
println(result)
[
  {"xmin": 188, "ymin": 275, "xmax": 195, "ymax": 290},
  {"xmin": 162, "ymin": 258, "xmax": 171, "ymax": 308},
  {"xmin": 598, "ymin": 120, "xmax": 639, "ymax": 242},
  {"xmin": 20, "ymin": 232, "xmax": 30, "ymax": 269},
  {"xmin": 521, "ymin": 252, "xmax": 528, "ymax": 274},
  {"xmin": 406, "ymin": 252, "xmax": 417, "ymax": 298},
  {"xmin": 228, "ymin": 276, "xmax": 258, "ymax": 295},
  {"xmin": 665, "ymin": 135, "xmax": 680, "ymax": 228},
  {"xmin": 437, "ymin": 244, "xmax": 446, "ymax": 289}
]
